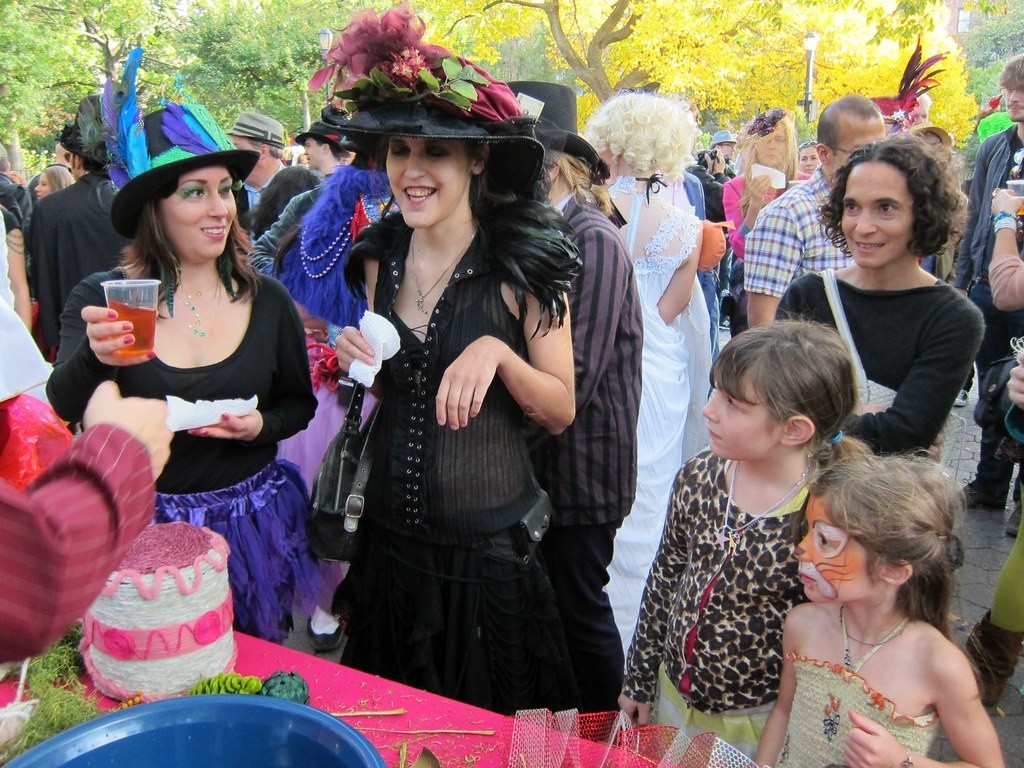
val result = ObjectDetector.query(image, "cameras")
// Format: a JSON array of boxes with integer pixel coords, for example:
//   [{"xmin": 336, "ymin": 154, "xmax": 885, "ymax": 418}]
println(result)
[{"xmin": 697, "ymin": 149, "xmax": 729, "ymax": 169}]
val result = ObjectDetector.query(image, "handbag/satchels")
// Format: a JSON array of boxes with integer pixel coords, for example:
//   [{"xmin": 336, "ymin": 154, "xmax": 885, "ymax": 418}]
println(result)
[
  {"xmin": 696, "ymin": 219, "xmax": 736, "ymax": 273},
  {"xmin": 719, "ymin": 288, "xmax": 739, "ymax": 317},
  {"xmin": 299, "ymin": 423, "xmax": 382, "ymax": 567},
  {"xmin": 728, "ymin": 257, "xmax": 745, "ymax": 284},
  {"xmin": 936, "ymin": 239, "xmax": 956, "ymax": 282},
  {"xmin": 841, "ymin": 373, "xmax": 965, "ymax": 472},
  {"xmin": 973, "ymin": 355, "xmax": 1019, "ymax": 428}
]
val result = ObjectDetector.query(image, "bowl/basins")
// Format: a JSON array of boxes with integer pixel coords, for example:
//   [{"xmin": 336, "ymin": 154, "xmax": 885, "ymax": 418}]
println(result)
[{"xmin": 0, "ymin": 693, "xmax": 387, "ymax": 768}]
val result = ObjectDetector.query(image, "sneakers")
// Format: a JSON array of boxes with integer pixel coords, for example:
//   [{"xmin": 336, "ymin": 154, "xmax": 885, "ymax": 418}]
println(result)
[{"xmin": 954, "ymin": 389, "xmax": 969, "ymax": 406}]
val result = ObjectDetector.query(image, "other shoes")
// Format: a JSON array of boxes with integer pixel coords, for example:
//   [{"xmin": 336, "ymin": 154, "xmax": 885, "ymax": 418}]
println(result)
[
  {"xmin": 306, "ymin": 613, "xmax": 344, "ymax": 653},
  {"xmin": 1005, "ymin": 499, "xmax": 1023, "ymax": 535},
  {"xmin": 952, "ymin": 481, "xmax": 1005, "ymax": 510}
]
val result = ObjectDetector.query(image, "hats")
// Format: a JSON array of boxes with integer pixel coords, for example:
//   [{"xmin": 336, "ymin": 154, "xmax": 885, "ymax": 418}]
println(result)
[
  {"xmin": 100, "ymin": 47, "xmax": 260, "ymax": 240},
  {"xmin": 225, "ymin": 111, "xmax": 286, "ymax": 150},
  {"xmin": 59, "ymin": 95, "xmax": 115, "ymax": 165},
  {"xmin": 499, "ymin": 81, "xmax": 601, "ymax": 169},
  {"xmin": 317, "ymin": 1, "xmax": 546, "ymax": 194},
  {"xmin": 870, "ymin": 36, "xmax": 950, "ymax": 124},
  {"xmin": 710, "ymin": 130, "xmax": 736, "ymax": 147},
  {"xmin": 294, "ymin": 120, "xmax": 350, "ymax": 155},
  {"xmin": 909, "ymin": 122, "xmax": 952, "ymax": 150}
]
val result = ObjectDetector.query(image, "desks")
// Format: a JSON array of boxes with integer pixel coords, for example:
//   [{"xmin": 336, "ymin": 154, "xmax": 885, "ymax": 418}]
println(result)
[{"xmin": 0, "ymin": 631, "xmax": 675, "ymax": 768}]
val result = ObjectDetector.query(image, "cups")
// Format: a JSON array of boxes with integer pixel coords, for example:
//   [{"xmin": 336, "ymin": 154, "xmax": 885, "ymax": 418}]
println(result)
[
  {"xmin": 1005, "ymin": 179, "xmax": 1024, "ymax": 216},
  {"xmin": 100, "ymin": 279, "xmax": 162, "ymax": 356}
]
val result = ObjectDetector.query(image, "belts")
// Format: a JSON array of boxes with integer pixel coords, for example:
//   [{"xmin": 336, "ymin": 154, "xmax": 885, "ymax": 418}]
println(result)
[{"xmin": 972, "ymin": 275, "xmax": 990, "ymax": 284}]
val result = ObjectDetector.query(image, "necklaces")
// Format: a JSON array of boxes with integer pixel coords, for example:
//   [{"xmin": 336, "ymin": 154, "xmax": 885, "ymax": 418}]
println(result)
[
  {"xmin": 713, "ymin": 448, "xmax": 813, "ymax": 550},
  {"xmin": 411, "ymin": 226, "xmax": 475, "ymax": 314},
  {"xmin": 178, "ymin": 275, "xmax": 221, "ymax": 337},
  {"xmin": 840, "ymin": 604, "xmax": 904, "ymax": 646}
]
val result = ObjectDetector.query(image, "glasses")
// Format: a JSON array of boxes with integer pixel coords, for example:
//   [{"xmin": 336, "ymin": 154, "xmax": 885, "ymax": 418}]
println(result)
[
  {"xmin": 799, "ymin": 141, "xmax": 817, "ymax": 148},
  {"xmin": 718, "ymin": 143, "xmax": 735, "ymax": 149}
]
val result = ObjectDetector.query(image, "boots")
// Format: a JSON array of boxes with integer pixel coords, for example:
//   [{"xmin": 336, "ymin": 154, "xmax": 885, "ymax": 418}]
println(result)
[{"xmin": 966, "ymin": 610, "xmax": 1024, "ymax": 708}]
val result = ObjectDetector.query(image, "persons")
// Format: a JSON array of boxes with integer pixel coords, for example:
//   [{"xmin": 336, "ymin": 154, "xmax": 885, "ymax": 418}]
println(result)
[
  {"xmin": 580, "ymin": 86, "xmax": 723, "ymax": 675},
  {"xmin": 709, "ymin": 91, "xmax": 974, "ymax": 406},
  {"xmin": 753, "ymin": 450, "xmax": 1005, "ymax": 767},
  {"xmin": 506, "ymin": 81, "xmax": 644, "ymax": 713},
  {"xmin": 222, "ymin": 105, "xmax": 398, "ymax": 654},
  {"xmin": 774, "ymin": 135, "xmax": 985, "ymax": 458},
  {"xmin": 0, "ymin": 95, "xmax": 175, "ymax": 666},
  {"xmin": 46, "ymin": 101, "xmax": 319, "ymax": 645},
  {"xmin": 334, "ymin": 43, "xmax": 584, "ymax": 719},
  {"xmin": 617, "ymin": 318, "xmax": 875, "ymax": 768},
  {"xmin": 953, "ymin": 53, "xmax": 1024, "ymax": 710}
]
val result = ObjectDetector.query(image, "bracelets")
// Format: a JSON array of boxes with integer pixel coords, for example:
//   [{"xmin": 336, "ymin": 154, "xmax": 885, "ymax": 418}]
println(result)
[
  {"xmin": 993, "ymin": 211, "xmax": 1018, "ymax": 234},
  {"xmin": 901, "ymin": 749, "xmax": 914, "ymax": 768}
]
